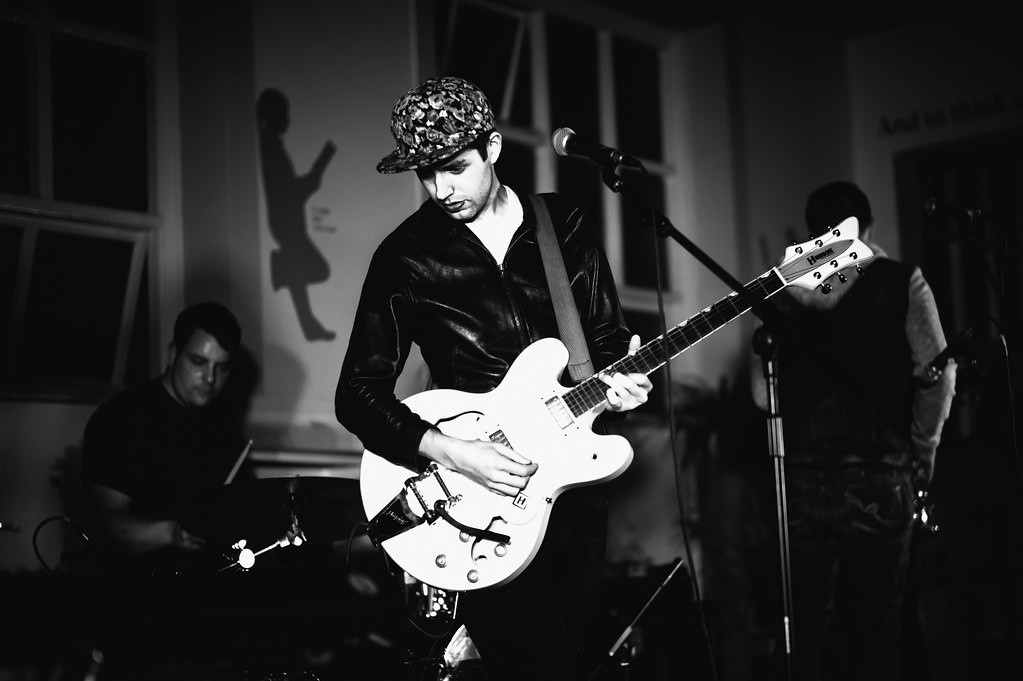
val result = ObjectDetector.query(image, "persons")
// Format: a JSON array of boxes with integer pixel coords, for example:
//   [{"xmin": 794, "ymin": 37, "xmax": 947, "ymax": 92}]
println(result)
[
  {"xmin": 334, "ymin": 75, "xmax": 654, "ymax": 681},
  {"xmin": 81, "ymin": 302, "xmax": 299, "ymax": 681},
  {"xmin": 749, "ymin": 181, "xmax": 958, "ymax": 681}
]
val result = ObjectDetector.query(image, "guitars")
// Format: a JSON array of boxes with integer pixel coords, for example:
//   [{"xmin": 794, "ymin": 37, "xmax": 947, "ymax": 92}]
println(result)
[{"xmin": 358, "ymin": 217, "xmax": 877, "ymax": 594}]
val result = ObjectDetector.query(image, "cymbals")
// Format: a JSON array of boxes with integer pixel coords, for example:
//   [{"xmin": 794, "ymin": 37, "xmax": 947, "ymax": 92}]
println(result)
[{"xmin": 178, "ymin": 476, "xmax": 370, "ymax": 544}]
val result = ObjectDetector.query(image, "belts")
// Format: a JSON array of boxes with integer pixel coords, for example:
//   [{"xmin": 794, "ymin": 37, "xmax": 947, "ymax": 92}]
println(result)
[{"xmin": 785, "ymin": 448, "xmax": 916, "ymax": 469}]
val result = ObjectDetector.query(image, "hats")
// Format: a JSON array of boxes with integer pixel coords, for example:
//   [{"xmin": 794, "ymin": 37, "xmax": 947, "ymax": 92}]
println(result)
[{"xmin": 376, "ymin": 75, "xmax": 496, "ymax": 174}]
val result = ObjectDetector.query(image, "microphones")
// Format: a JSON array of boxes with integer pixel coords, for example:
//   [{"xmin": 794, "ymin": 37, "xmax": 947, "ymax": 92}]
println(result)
[
  {"xmin": 549, "ymin": 124, "xmax": 644, "ymax": 172},
  {"xmin": 921, "ymin": 324, "xmax": 976, "ymax": 385}
]
